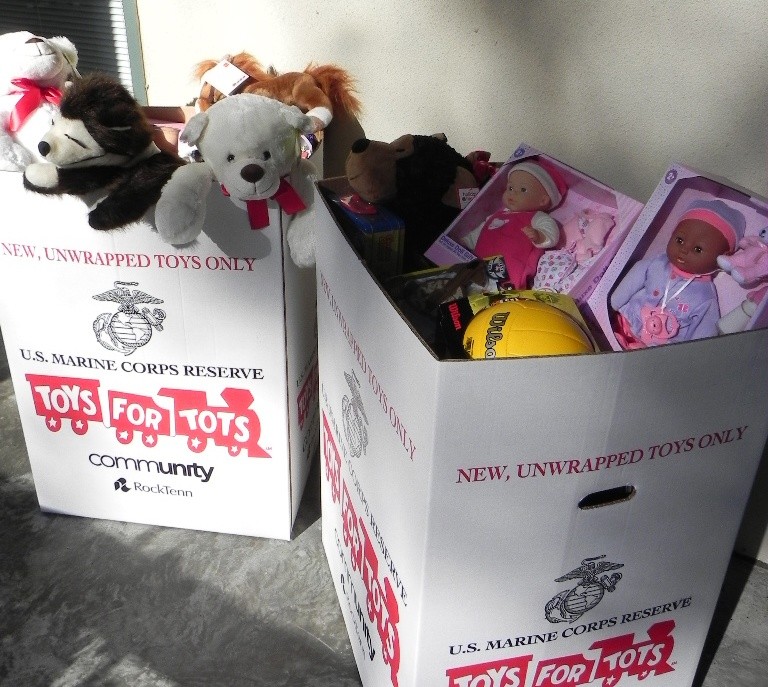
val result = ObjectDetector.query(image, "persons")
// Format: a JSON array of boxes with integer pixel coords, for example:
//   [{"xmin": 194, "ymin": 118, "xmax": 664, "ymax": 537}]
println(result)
[
  {"xmin": 609, "ymin": 200, "xmax": 747, "ymax": 346},
  {"xmin": 456, "ymin": 160, "xmax": 568, "ymax": 290}
]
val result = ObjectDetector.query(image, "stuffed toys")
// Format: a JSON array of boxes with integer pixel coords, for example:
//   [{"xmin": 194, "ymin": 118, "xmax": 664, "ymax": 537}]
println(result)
[
  {"xmin": 155, "ymin": 92, "xmax": 316, "ymax": 268},
  {"xmin": 0, "ymin": 31, "xmax": 78, "ymax": 172},
  {"xmin": 191, "ymin": 50, "xmax": 363, "ymax": 132},
  {"xmin": 717, "ymin": 226, "xmax": 768, "ymax": 286},
  {"xmin": 345, "ymin": 132, "xmax": 480, "ymax": 271},
  {"xmin": 23, "ymin": 69, "xmax": 188, "ymax": 232}
]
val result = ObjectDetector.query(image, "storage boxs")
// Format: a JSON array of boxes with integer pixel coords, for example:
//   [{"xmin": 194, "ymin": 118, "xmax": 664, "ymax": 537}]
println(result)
[
  {"xmin": 585, "ymin": 163, "xmax": 768, "ymax": 352},
  {"xmin": 330, "ymin": 195, "xmax": 405, "ymax": 276},
  {"xmin": 1, "ymin": 107, "xmax": 317, "ymax": 542},
  {"xmin": 424, "ymin": 142, "xmax": 644, "ymax": 310},
  {"xmin": 313, "ymin": 161, "xmax": 768, "ymax": 687}
]
